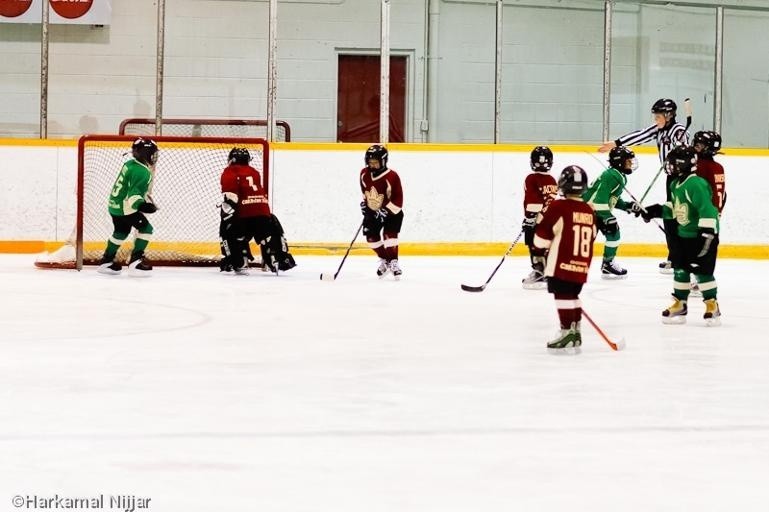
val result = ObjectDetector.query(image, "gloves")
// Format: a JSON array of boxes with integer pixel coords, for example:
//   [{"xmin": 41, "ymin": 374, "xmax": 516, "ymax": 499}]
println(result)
[
  {"xmin": 603, "ymin": 216, "xmax": 620, "ymax": 236},
  {"xmin": 522, "ymin": 213, "xmax": 536, "ymax": 232},
  {"xmin": 625, "ymin": 200, "xmax": 640, "ymax": 217}
]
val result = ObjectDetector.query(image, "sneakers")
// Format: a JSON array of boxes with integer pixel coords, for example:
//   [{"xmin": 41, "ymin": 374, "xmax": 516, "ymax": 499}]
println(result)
[{"xmin": 659, "ymin": 259, "xmax": 672, "ymax": 270}]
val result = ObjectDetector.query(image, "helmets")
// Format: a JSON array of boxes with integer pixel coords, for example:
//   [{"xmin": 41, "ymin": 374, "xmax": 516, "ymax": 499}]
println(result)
[
  {"xmin": 132, "ymin": 137, "xmax": 159, "ymax": 167},
  {"xmin": 530, "ymin": 146, "xmax": 554, "ymax": 171},
  {"xmin": 364, "ymin": 145, "xmax": 389, "ymax": 172},
  {"xmin": 227, "ymin": 147, "xmax": 253, "ymax": 165},
  {"xmin": 651, "ymin": 98, "xmax": 677, "ymax": 131},
  {"xmin": 663, "ymin": 145, "xmax": 698, "ymax": 178},
  {"xmin": 693, "ymin": 131, "xmax": 725, "ymax": 156},
  {"xmin": 558, "ymin": 165, "xmax": 586, "ymax": 194},
  {"xmin": 609, "ymin": 146, "xmax": 639, "ymax": 175}
]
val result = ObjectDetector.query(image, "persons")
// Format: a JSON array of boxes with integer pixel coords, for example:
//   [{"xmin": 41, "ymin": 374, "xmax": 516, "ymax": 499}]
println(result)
[
  {"xmin": 521, "ymin": 145, "xmax": 558, "ymax": 285},
  {"xmin": 531, "ymin": 166, "xmax": 598, "ymax": 349},
  {"xmin": 360, "ymin": 145, "xmax": 402, "ymax": 276},
  {"xmin": 583, "ymin": 145, "xmax": 640, "ymax": 275},
  {"xmin": 640, "ymin": 144, "xmax": 722, "ymax": 320},
  {"xmin": 218, "ymin": 148, "xmax": 297, "ymax": 275},
  {"xmin": 598, "ymin": 97, "xmax": 693, "ymax": 270},
  {"xmin": 689, "ymin": 129, "xmax": 726, "ymax": 291},
  {"xmin": 97, "ymin": 136, "xmax": 159, "ymax": 274}
]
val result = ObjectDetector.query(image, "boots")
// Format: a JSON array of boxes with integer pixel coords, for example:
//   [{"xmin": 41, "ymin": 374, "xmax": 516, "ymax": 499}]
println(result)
[
  {"xmin": 703, "ymin": 297, "xmax": 722, "ymax": 320},
  {"xmin": 389, "ymin": 259, "xmax": 403, "ymax": 276},
  {"xmin": 547, "ymin": 327, "xmax": 581, "ymax": 349},
  {"xmin": 662, "ymin": 298, "xmax": 690, "ymax": 318},
  {"xmin": 128, "ymin": 257, "xmax": 152, "ymax": 271},
  {"xmin": 521, "ymin": 267, "xmax": 548, "ymax": 284},
  {"xmin": 98, "ymin": 258, "xmax": 121, "ymax": 271},
  {"xmin": 601, "ymin": 258, "xmax": 627, "ymax": 276},
  {"xmin": 376, "ymin": 259, "xmax": 388, "ymax": 276}
]
